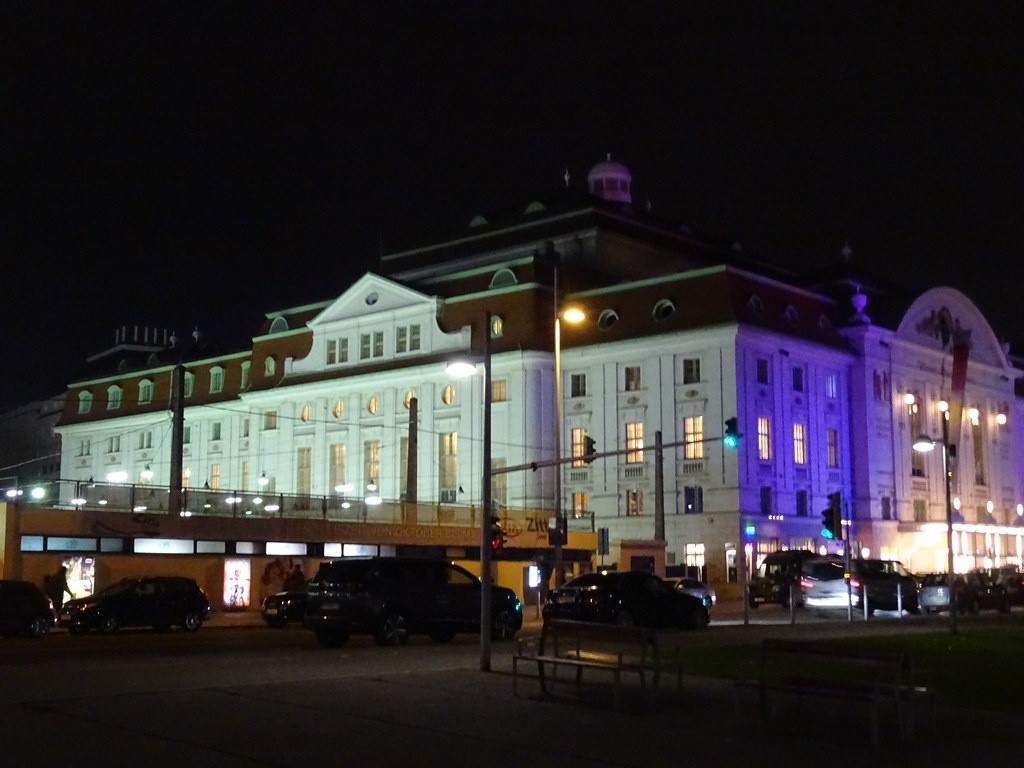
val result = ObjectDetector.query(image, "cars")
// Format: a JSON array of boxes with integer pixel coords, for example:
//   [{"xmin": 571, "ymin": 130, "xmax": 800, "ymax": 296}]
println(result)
[
  {"xmin": 542, "ymin": 570, "xmax": 710, "ymax": 632},
  {"xmin": 260, "ymin": 576, "xmax": 314, "ymax": 628},
  {"xmin": 749, "ymin": 549, "xmax": 1024, "ymax": 616},
  {"xmin": 0, "ymin": 579, "xmax": 58, "ymax": 639},
  {"xmin": 663, "ymin": 575, "xmax": 717, "ymax": 610}
]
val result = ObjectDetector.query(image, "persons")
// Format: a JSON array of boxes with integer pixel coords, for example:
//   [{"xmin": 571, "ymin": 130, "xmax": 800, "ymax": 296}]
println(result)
[
  {"xmin": 290, "ymin": 565, "xmax": 304, "ymax": 589},
  {"xmin": 46, "ymin": 566, "xmax": 75, "ymax": 628}
]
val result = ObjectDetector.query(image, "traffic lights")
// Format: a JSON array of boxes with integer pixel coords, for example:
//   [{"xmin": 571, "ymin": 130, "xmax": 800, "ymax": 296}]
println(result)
[
  {"xmin": 583, "ymin": 436, "xmax": 596, "ymax": 463},
  {"xmin": 725, "ymin": 417, "xmax": 738, "ymax": 448},
  {"xmin": 490, "ymin": 528, "xmax": 504, "ymax": 561},
  {"xmin": 822, "ymin": 492, "xmax": 843, "ymax": 542}
]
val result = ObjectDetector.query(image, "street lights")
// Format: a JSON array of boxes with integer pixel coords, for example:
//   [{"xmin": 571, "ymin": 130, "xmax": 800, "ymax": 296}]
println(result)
[
  {"xmin": 912, "ymin": 409, "xmax": 956, "ymax": 633},
  {"xmin": 554, "ymin": 298, "xmax": 587, "ymax": 588}
]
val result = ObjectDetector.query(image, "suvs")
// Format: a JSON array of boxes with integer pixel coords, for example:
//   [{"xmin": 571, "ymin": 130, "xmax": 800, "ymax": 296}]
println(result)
[
  {"xmin": 62, "ymin": 574, "xmax": 212, "ymax": 637},
  {"xmin": 303, "ymin": 555, "xmax": 522, "ymax": 650}
]
